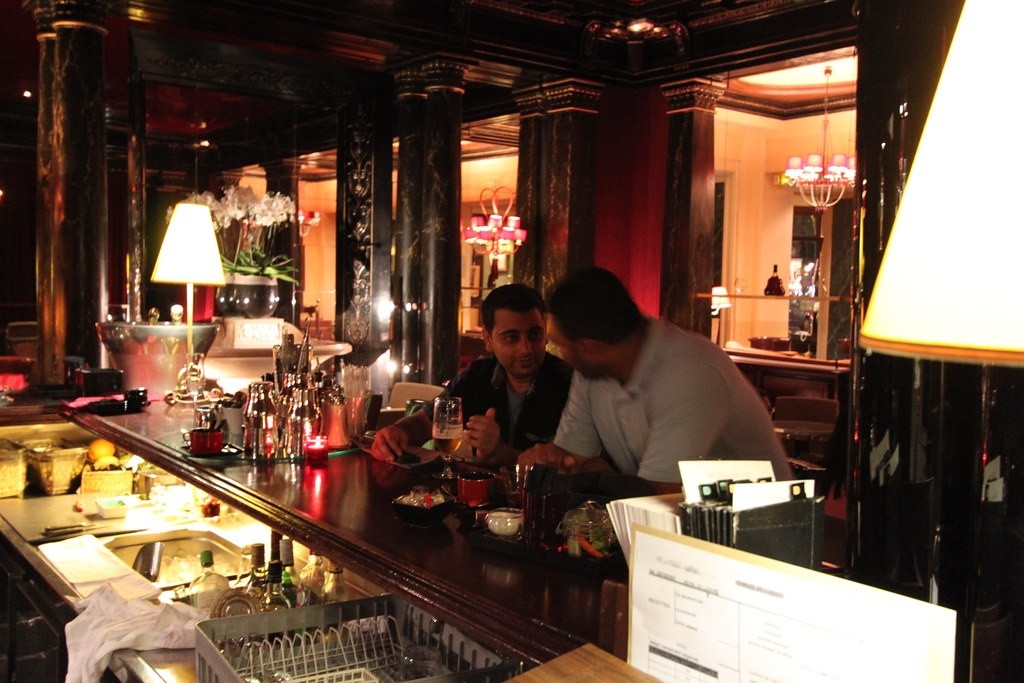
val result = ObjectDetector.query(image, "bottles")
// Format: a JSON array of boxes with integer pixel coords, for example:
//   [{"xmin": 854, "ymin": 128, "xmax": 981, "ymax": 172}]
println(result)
[
  {"xmin": 214, "ymin": 317, "xmax": 382, "ymax": 464},
  {"xmin": 173, "ymin": 529, "xmax": 350, "ymax": 656},
  {"xmin": 764, "ymin": 265, "xmax": 785, "ymax": 294},
  {"xmin": 487, "ymin": 259, "xmax": 498, "ymax": 287},
  {"xmin": 106, "ymin": 304, "xmax": 184, "ymax": 323},
  {"xmin": 555, "ymin": 500, "xmax": 614, "ymax": 557}
]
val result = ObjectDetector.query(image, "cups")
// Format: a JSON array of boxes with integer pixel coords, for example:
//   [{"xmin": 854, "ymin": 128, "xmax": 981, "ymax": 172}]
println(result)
[
  {"xmin": 182, "ymin": 427, "xmax": 225, "ymax": 454},
  {"xmin": 303, "ymin": 435, "xmax": 328, "ymax": 464},
  {"xmin": 186, "ymin": 353, "xmax": 205, "ymax": 405},
  {"xmin": 402, "ymin": 644, "xmax": 441, "ymax": 681},
  {"xmin": 500, "ymin": 463, "xmax": 532, "ymax": 504},
  {"xmin": 457, "ymin": 472, "xmax": 501, "ymax": 507}
]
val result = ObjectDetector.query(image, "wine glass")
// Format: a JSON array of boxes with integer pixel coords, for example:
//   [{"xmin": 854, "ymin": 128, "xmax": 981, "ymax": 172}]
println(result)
[{"xmin": 431, "ymin": 397, "xmax": 464, "ymax": 479}]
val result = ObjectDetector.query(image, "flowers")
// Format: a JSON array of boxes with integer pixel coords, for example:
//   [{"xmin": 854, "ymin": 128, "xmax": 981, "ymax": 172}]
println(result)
[
  {"xmin": 165, "ymin": 190, "xmax": 235, "ymax": 274},
  {"xmin": 213, "ymin": 185, "xmax": 300, "ymax": 286}
]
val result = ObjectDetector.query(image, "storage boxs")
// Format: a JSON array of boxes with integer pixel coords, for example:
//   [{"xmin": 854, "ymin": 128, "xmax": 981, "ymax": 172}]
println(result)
[{"xmin": 81, "ymin": 464, "xmax": 133, "ymax": 495}]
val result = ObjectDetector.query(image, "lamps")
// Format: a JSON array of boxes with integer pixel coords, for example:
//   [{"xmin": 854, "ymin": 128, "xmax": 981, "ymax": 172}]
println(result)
[
  {"xmin": 150, "ymin": 202, "xmax": 225, "ymax": 405},
  {"xmin": 858, "ymin": 0, "xmax": 1024, "ymax": 368},
  {"xmin": 299, "ymin": 210, "xmax": 320, "ymax": 237},
  {"xmin": 784, "ymin": 65, "xmax": 857, "ymax": 212},
  {"xmin": 463, "ymin": 186, "xmax": 527, "ymax": 255}
]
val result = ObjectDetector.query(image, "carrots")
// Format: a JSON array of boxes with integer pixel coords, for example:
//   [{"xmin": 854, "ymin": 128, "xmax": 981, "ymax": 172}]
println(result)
[{"xmin": 576, "ymin": 534, "xmax": 604, "ymax": 559}]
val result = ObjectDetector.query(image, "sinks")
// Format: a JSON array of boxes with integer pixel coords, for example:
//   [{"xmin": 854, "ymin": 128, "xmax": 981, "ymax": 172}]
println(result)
[{"xmin": 108, "ymin": 537, "xmax": 251, "ymax": 591}]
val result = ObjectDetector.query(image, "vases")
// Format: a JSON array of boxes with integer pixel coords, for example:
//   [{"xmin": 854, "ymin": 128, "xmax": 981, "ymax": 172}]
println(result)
[
  {"xmin": 234, "ymin": 272, "xmax": 280, "ymax": 317},
  {"xmin": 216, "ymin": 274, "xmax": 240, "ymax": 317}
]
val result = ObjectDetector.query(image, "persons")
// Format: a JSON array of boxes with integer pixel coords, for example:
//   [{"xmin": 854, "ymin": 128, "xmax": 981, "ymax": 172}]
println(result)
[
  {"xmin": 518, "ymin": 268, "xmax": 794, "ymax": 496},
  {"xmin": 370, "ymin": 283, "xmax": 618, "ymax": 474}
]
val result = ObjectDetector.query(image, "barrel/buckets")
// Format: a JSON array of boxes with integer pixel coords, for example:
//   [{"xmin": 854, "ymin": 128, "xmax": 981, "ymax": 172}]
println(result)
[{"xmin": 97, "ymin": 322, "xmax": 217, "ymax": 402}]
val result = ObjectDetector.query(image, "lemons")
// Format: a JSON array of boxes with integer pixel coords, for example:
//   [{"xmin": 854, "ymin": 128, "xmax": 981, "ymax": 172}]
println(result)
[{"xmin": 87, "ymin": 438, "xmax": 132, "ymax": 470}]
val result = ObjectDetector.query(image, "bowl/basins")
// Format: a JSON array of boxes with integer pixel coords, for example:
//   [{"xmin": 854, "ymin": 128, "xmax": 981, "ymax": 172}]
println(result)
[
  {"xmin": 391, "ymin": 494, "xmax": 457, "ymax": 532},
  {"xmin": 485, "ymin": 507, "xmax": 528, "ymax": 540}
]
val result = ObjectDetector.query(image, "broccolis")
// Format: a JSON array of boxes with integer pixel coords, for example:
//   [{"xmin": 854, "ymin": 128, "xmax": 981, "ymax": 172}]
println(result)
[{"xmin": 566, "ymin": 512, "xmax": 611, "ymax": 559}]
